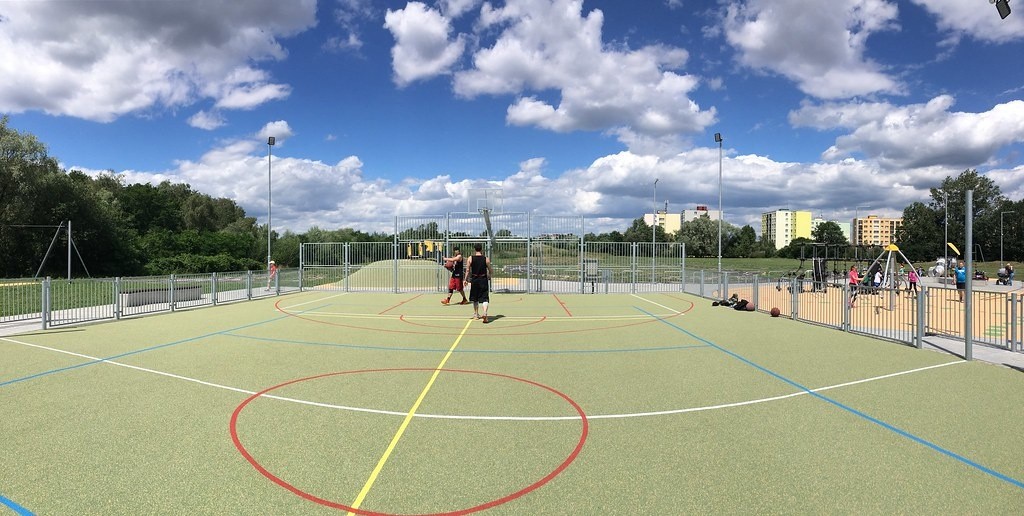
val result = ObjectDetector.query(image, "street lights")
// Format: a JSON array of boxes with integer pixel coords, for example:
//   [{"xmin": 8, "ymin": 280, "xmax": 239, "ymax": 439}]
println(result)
[
  {"xmin": 713, "ymin": 133, "xmax": 724, "ymax": 299},
  {"xmin": 1000, "ymin": 211, "xmax": 1016, "ymax": 268},
  {"xmin": 855, "ymin": 205, "xmax": 871, "ymax": 244},
  {"xmin": 266, "ymin": 136, "xmax": 277, "ymax": 289},
  {"xmin": 942, "ymin": 191, "xmax": 947, "ymax": 289},
  {"xmin": 652, "ymin": 178, "xmax": 659, "ymax": 283}
]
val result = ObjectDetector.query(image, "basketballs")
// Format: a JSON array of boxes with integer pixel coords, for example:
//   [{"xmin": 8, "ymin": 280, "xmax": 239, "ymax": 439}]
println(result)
[
  {"xmin": 446, "ymin": 262, "xmax": 453, "ymax": 270},
  {"xmin": 746, "ymin": 303, "xmax": 755, "ymax": 310},
  {"xmin": 771, "ymin": 308, "xmax": 780, "ymax": 316}
]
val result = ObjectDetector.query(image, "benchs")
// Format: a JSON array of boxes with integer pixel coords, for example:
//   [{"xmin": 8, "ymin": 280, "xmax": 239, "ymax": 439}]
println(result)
[{"xmin": 117, "ymin": 284, "xmax": 201, "ymax": 307}]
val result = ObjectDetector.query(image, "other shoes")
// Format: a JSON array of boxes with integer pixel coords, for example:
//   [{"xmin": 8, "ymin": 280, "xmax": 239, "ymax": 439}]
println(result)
[
  {"xmin": 474, "ymin": 314, "xmax": 479, "ymax": 319},
  {"xmin": 482, "ymin": 314, "xmax": 489, "ymax": 323},
  {"xmin": 460, "ymin": 300, "xmax": 468, "ymax": 304},
  {"xmin": 441, "ymin": 299, "xmax": 450, "ymax": 305}
]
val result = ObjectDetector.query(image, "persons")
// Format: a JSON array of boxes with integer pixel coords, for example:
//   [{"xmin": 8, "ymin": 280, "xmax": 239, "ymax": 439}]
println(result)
[
  {"xmin": 874, "ymin": 270, "xmax": 883, "ymax": 294},
  {"xmin": 440, "ymin": 247, "xmax": 469, "ymax": 305},
  {"xmin": 899, "ymin": 261, "xmax": 905, "ymax": 276},
  {"xmin": 951, "ymin": 255, "xmax": 957, "ymax": 268},
  {"xmin": 1006, "ymin": 262, "xmax": 1015, "ymax": 286},
  {"xmin": 264, "ymin": 260, "xmax": 277, "ymax": 291},
  {"xmin": 953, "ymin": 261, "xmax": 966, "ymax": 303},
  {"xmin": 848, "ymin": 265, "xmax": 863, "ymax": 308},
  {"xmin": 463, "ymin": 243, "xmax": 492, "ymax": 323},
  {"xmin": 908, "ymin": 266, "xmax": 917, "ymax": 296}
]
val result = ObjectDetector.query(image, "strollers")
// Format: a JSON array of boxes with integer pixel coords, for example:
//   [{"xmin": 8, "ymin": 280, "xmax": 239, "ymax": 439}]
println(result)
[{"xmin": 996, "ymin": 273, "xmax": 1010, "ymax": 285}]
